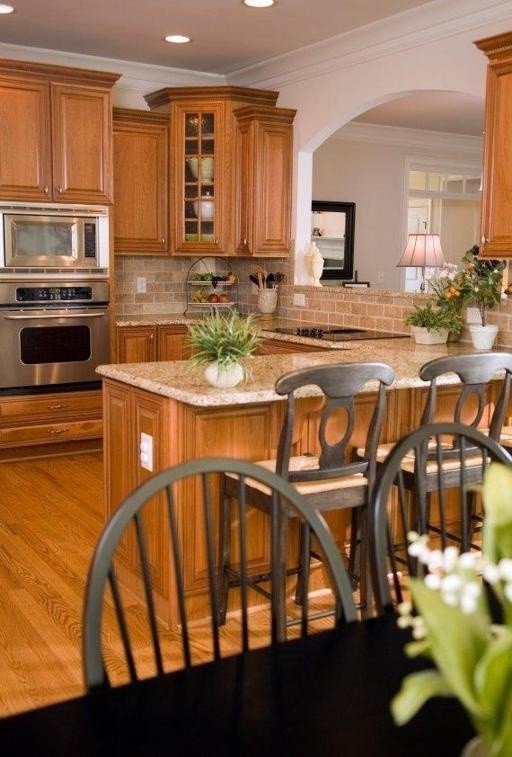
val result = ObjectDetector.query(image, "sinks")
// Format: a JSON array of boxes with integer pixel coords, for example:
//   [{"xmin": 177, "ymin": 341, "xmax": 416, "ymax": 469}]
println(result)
[{"xmin": 324, "ymin": 328, "xmax": 365, "ymax": 334}]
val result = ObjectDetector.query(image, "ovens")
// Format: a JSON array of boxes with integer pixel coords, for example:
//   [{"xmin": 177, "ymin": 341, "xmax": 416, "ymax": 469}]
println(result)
[{"xmin": 1, "ymin": 280, "xmax": 110, "ymax": 393}]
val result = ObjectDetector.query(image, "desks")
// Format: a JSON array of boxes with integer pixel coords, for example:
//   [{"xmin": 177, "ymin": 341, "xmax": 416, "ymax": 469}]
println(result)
[{"xmin": 0, "ymin": 609, "xmax": 509, "ymax": 757}]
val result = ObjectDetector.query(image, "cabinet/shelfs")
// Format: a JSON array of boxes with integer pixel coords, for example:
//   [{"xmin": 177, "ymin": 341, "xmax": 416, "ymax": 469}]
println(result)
[
  {"xmin": 472, "ymin": 30, "xmax": 512, "ymax": 259},
  {"xmin": 113, "ymin": 107, "xmax": 171, "ymax": 254},
  {"xmin": 1, "ymin": 57, "xmax": 122, "ymax": 204},
  {"xmin": 0, "ymin": 388, "xmax": 105, "ymax": 450},
  {"xmin": 145, "ymin": 87, "xmax": 296, "ymax": 258},
  {"xmin": 115, "ymin": 324, "xmax": 196, "ymax": 365}
]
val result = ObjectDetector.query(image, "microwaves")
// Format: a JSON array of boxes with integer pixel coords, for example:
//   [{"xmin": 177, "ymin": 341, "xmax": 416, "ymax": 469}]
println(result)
[{"xmin": 4, "ymin": 214, "xmax": 99, "ymax": 267}]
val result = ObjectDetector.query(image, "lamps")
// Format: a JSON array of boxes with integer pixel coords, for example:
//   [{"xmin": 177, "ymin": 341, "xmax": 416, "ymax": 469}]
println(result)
[{"xmin": 396, "ymin": 235, "xmax": 445, "ymax": 292}]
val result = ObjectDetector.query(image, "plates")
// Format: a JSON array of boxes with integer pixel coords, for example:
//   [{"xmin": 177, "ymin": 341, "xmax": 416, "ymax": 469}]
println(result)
[
  {"xmin": 188, "ymin": 280, "xmax": 235, "ymax": 286},
  {"xmin": 185, "ymin": 233, "xmax": 214, "ymax": 240},
  {"xmin": 189, "ymin": 303, "xmax": 234, "ymax": 307}
]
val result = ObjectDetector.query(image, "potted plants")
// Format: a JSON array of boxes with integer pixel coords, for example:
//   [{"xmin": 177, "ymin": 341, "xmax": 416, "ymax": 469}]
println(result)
[
  {"xmin": 404, "ymin": 306, "xmax": 462, "ymax": 344},
  {"xmin": 187, "ymin": 306, "xmax": 263, "ymax": 388},
  {"xmin": 434, "ymin": 263, "xmax": 511, "ymax": 349}
]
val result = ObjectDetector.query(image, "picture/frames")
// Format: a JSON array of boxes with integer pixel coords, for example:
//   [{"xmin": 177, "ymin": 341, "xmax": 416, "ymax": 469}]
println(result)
[{"xmin": 311, "ymin": 200, "xmax": 356, "ymax": 280}]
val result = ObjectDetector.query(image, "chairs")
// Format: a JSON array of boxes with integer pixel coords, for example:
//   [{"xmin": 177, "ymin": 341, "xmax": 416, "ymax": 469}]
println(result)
[
  {"xmin": 355, "ymin": 352, "xmax": 512, "ymax": 606},
  {"xmin": 212, "ymin": 362, "xmax": 394, "ymax": 627},
  {"xmin": 82, "ymin": 458, "xmax": 358, "ymax": 692},
  {"xmin": 369, "ymin": 424, "xmax": 512, "ymax": 616}
]
